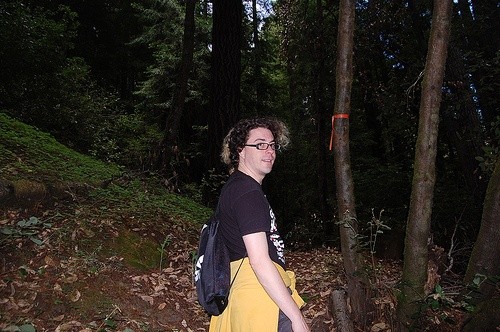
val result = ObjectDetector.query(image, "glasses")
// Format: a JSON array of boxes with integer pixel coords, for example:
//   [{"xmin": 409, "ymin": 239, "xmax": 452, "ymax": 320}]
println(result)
[{"xmin": 244, "ymin": 143, "xmax": 281, "ymax": 150}]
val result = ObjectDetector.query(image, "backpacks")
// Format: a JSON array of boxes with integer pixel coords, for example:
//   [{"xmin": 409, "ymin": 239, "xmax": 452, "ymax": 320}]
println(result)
[{"xmin": 194, "ymin": 177, "xmax": 260, "ymax": 316}]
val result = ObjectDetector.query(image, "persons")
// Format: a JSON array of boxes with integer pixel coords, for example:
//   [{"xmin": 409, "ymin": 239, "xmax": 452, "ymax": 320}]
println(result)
[{"xmin": 209, "ymin": 116, "xmax": 311, "ymax": 332}]
